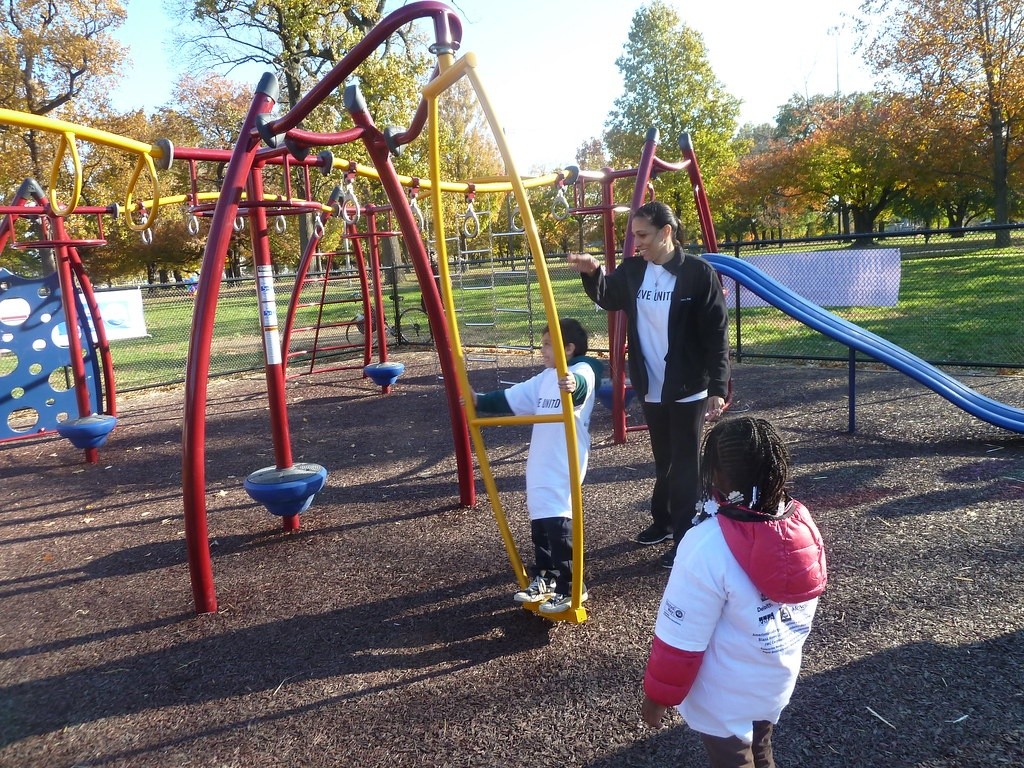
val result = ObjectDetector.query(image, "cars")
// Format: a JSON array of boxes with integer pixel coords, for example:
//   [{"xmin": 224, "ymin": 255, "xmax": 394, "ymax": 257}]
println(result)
[{"xmin": 75, "ymin": 284, "xmax": 115, "ymax": 293}]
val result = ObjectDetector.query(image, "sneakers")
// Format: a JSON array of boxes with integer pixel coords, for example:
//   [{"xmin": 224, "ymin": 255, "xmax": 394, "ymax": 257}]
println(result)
[
  {"xmin": 538, "ymin": 592, "xmax": 589, "ymax": 613},
  {"xmin": 657, "ymin": 547, "xmax": 677, "ymax": 568},
  {"xmin": 513, "ymin": 574, "xmax": 557, "ymax": 602},
  {"xmin": 634, "ymin": 522, "xmax": 674, "ymax": 544}
]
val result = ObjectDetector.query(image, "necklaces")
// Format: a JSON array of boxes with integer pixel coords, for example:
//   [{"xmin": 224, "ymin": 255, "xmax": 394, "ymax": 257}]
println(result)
[{"xmin": 652, "ymin": 266, "xmax": 665, "ymax": 288}]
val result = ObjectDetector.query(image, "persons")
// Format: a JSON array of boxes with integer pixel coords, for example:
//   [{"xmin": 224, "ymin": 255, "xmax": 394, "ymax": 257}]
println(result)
[
  {"xmin": 458, "ymin": 319, "xmax": 603, "ymax": 614},
  {"xmin": 423, "ymin": 252, "xmax": 441, "ymax": 306},
  {"xmin": 642, "ymin": 416, "xmax": 827, "ymax": 768},
  {"xmin": 569, "ymin": 202, "xmax": 731, "ymax": 568}
]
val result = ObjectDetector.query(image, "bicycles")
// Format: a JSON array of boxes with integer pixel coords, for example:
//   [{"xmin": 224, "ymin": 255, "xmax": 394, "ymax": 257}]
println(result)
[{"xmin": 344, "ymin": 291, "xmax": 433, "ymax": 348}]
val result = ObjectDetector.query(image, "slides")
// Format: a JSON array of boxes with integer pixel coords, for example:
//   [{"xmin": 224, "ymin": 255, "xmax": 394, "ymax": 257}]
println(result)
[{"xmin": 700, "ymin": 251, "xmax": 1024, "ymax": 433}]
